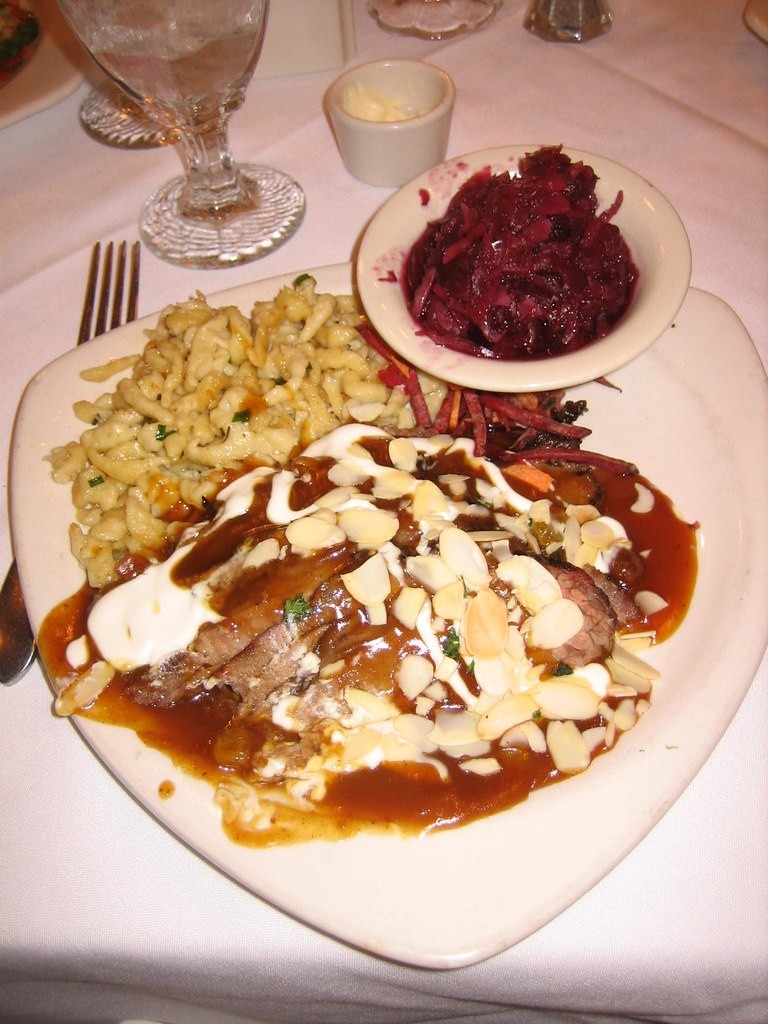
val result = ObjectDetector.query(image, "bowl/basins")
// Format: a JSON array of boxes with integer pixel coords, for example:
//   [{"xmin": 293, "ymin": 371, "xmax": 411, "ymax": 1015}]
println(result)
[{"xmin": 322, "ymin": 57, "xmax": 457, "ymax": 189}]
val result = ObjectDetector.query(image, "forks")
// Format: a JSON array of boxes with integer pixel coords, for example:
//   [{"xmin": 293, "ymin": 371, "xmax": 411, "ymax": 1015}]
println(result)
[{"xmin": 0, "ymin": 241, "xmax": 142, "ymax": 686}]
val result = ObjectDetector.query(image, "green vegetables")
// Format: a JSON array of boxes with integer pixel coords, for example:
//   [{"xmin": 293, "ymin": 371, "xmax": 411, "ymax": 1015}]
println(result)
[{"xmin": 88, "ymin": 272, "xmax": 575, "ymax": 723}]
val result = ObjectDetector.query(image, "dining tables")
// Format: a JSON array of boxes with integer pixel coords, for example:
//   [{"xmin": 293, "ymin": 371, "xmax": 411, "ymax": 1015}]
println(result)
[{"xmin": 1, "ymin": 2, "xmax": 768, "ymax": 1024}]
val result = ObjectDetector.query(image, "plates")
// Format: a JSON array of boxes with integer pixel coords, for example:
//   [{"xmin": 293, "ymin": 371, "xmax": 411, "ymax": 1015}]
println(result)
[
  {"xmin": 744, "ymin": 0, "xmax": 768, "ymax": 43},
  {"xmin": 356, "ymin": 144, "xmax": 691, "ymax": 393},
  {"xmin": 0, "ymin": 264, "xmax": 768, "ymax": 970},
  {"xmin": 0, "ymin": 0, "xmax": 89, "ymax": 132}
]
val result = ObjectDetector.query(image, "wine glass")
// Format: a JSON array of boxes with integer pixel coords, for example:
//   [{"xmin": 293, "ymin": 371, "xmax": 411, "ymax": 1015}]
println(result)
[
  {"xmin": 56, "ymin": 0, "xmax": 306, "ymax": 270},
  {"xmin": 80, "ymin": 81, "xmax": 244, "ymax": 151}
]
